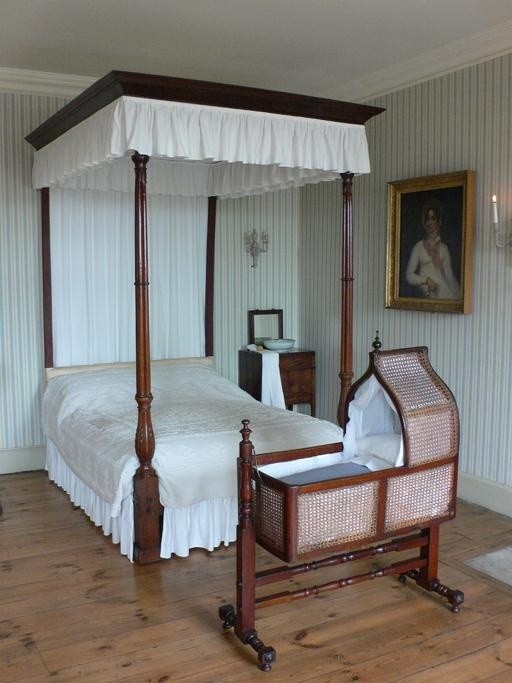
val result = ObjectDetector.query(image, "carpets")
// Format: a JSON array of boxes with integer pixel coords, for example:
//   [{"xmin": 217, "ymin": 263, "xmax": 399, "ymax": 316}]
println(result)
[{"xmin": 462, "ymin": 542, "xmax": 511, "ymax": 587}]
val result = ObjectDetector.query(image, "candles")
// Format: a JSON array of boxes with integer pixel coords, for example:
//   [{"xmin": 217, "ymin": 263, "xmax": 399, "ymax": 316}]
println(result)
[{"xmin": 490, "ymin": 193, "xmax": 498, "ymax": 223}]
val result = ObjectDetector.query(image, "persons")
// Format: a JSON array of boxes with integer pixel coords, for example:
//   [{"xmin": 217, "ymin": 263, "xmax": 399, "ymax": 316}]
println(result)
[{"xmin": 405, "ymin": 207, "xmax": 460, "ymax": 299}]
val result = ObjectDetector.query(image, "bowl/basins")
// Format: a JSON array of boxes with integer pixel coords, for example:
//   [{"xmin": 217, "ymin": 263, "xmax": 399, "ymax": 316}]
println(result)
[
  {"xmin": 255, "ymin": 337, "xmax": 271, "ymax": 346},
  {"xmin": 263, "ymin": 339, "xmax": 296, "ymax": 353}
]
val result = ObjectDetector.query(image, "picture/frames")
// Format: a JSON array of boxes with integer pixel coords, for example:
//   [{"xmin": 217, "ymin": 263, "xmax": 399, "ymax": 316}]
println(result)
[{"xmin": 384, "ymin": 168, "xmax": 477, "ymax": 316}]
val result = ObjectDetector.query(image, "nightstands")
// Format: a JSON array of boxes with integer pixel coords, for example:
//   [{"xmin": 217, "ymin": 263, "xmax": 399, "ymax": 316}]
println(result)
[{"xmin": 238, "ymin": 350, "xmax": 316, "ymax": 418}]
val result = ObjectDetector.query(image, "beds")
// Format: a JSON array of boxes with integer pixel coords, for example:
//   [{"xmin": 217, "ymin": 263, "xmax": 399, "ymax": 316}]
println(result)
[
  {"xmin": 214, "ymin": 346, "xmax": 461, "ymax": 667},
  {"xmin": 45, "ymin": 355, "xmax": 344, "ymax": 560}
]
